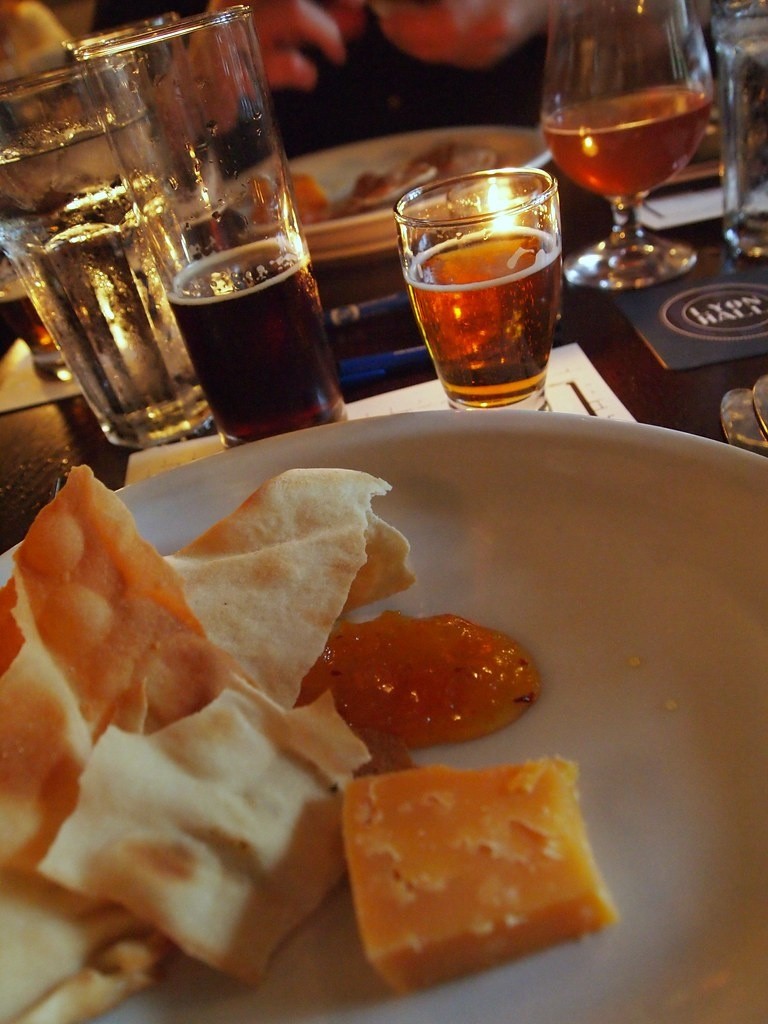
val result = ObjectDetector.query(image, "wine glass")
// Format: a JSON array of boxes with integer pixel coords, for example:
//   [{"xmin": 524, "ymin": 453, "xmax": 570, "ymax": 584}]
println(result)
[{"xmin": 537, "ymin": 0, "xmax": 716, "ymax": 292}]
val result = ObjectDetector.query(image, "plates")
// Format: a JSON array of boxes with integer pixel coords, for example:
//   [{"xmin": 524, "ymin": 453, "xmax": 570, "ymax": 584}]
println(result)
[
  {"xmin": 232, "ymin": 122, "xmax": 564, "ymax": 263},
  {"xmin": 0, "ymin": 407, "xmax": 768, "ymax": 1024}
]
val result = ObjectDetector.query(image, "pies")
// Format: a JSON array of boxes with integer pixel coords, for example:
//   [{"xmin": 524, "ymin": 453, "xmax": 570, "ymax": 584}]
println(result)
[{"xmin": 0, "ymin": 465, "xmax": 415, "ymax": 1024}]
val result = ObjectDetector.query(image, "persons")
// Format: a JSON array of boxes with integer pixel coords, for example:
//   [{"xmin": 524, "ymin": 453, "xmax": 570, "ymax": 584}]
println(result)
[{"xmin": 89, "ymin": 0, "xmax": 554, "ymax": 181}]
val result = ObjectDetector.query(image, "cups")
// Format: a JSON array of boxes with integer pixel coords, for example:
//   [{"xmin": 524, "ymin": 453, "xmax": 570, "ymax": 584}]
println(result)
[
  {"xmin": 390, "ymin": 168, "xmax": 562, "ymax": 422},
  {"xmin": 0, "ymin": 259, "xmax": 60, "ymax": 364},
  {"xmin": 70, "ymin": 0, "xmax": 351, "ymax": 442},
  {"xmin": 0, "ymin": 55, "xmax": 225, "ymax": 446}
]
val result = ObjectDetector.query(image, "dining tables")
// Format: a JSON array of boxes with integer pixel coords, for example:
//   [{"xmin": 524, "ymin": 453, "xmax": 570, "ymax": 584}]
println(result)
[{"xmin": 1, "ymin": 26, "xmax": 764, "ymax": 518}]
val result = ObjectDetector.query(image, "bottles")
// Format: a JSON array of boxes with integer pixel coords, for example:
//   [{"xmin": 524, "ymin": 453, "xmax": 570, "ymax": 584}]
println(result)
[{"xmin": 715, "ymin": 1, "xmax": 768, "ymax": 253}]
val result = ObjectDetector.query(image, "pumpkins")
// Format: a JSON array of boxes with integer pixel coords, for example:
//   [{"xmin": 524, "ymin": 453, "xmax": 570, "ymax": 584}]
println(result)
[{"xmin": 341, "ymin": 754, "xmax": 615, "ymax": 997}]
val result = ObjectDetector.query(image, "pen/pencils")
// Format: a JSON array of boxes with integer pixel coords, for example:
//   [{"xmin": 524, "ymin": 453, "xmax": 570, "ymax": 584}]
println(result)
[
  {"xmin": 325, "ymin": 291, "xmax": 408, "ymax": 329},
  {"xmin": 339, "ymin": 344, "xmax": 430, "ymax": 395}
]
